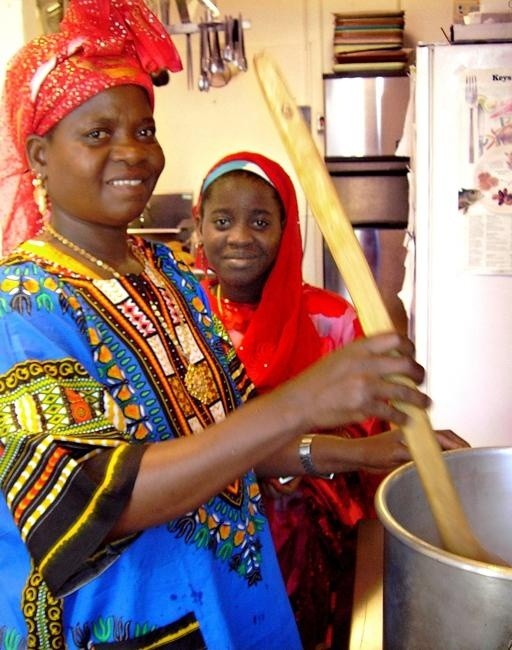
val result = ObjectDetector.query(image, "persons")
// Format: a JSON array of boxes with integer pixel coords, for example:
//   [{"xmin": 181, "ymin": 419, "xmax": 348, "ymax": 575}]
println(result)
[
  {"xmin": 0, "ymin": 31, "xmax": 470, "ymax": 650},
  {"xmin": 191, "ymin": 152, "xmax": 391, "ymax": 650}
]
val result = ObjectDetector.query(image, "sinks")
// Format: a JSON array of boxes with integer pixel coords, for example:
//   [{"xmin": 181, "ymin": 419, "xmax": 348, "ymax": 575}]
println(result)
[{"xmin": 124, "ymin": 193, "xmax": 194, "ymax": 244}]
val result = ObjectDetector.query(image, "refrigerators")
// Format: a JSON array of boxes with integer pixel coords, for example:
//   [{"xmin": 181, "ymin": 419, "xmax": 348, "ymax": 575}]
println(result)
[{"xmin": 412, "ymin": 43, "xmax": 512, "ymax": 451}]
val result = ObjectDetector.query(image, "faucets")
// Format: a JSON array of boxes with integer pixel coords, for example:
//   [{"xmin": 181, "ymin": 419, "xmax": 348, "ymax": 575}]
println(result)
[{"xmin": 138, "ymin": 203, "xmax": 155, "ymax": 226}]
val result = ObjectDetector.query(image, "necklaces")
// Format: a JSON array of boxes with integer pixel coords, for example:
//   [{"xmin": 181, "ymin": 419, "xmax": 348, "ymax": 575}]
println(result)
[
  {"xmin": 216, "ymin": 281, "xmax": 222, "ymax": 315},
  {"xmin": 41, "ymin": 221, "xmax": 217, "ymax": 405}
]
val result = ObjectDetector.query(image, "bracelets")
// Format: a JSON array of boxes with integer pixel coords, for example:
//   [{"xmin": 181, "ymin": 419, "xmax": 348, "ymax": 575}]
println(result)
[{"xmin": 299, "ymin": 433, "xmax": 334, "ymax": 481}]
